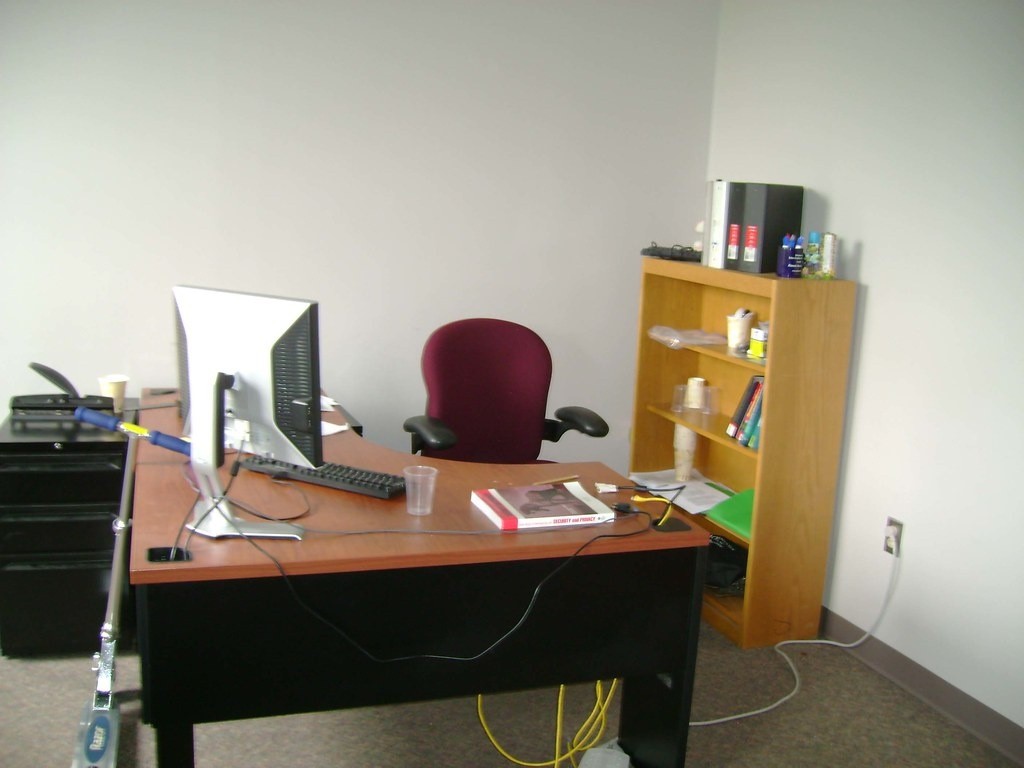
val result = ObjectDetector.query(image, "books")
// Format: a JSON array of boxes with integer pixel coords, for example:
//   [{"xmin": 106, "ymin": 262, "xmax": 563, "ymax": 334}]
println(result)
[
  {"xmin": 725, "ymin": 376, "xmax": 765, "ymax": 450},
  {"xmin": 469, "ymin": 480, "xmax": 615, "ymax": 531}
]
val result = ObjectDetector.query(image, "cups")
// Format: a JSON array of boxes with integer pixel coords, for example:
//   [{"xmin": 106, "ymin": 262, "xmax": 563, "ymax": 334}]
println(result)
[
  {"xmin": 701, "ymin": 387, "xmax": 717, "ymax": 416},
  {"xmin": 684, "ymin": 378, "xmax": 705, "ymax": 409},
  {"xmin": 777, "ymin": 248, "xmax": 803, "ymax": 278},
  {"xmin": 727, "ymin": 316, "xmax": 751, "ymax": 348},
  {"xmin": 99, "ymin": 375, "xmax": 129, "ymax": 414},
  {"xmin": 673, "ymin": 424, "xmax": 696, "ymax": 480},
  {"xmin": 403, "ymin": 466, "xmax": 438, "ymax": 515},
  {"xmin": 672, "ymin": 386, "xmax": 689, "ymax": 413}
]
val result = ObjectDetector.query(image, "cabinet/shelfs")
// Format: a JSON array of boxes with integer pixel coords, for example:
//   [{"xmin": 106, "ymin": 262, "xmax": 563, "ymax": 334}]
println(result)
[{"xmin": 626, "ymin": 179, "xmax": 856, "ymax": 646}]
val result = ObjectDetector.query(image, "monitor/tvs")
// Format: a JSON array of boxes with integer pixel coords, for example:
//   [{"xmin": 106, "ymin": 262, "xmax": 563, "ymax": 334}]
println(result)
[{"xmin": 172, "ymin": 286, "xmax": 324, "ymax": 538}]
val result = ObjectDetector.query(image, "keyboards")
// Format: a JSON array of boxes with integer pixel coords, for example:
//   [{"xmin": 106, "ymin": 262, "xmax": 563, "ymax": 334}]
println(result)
[{"xmin": 241, "ymin": 455, "xmax": 406, "ymax": 499}]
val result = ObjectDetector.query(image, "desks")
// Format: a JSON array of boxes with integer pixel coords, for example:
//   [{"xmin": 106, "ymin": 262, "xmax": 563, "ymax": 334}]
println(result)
[{"xmin": 128, "ymin": 383, "xmax": 710, "ymax": 768}]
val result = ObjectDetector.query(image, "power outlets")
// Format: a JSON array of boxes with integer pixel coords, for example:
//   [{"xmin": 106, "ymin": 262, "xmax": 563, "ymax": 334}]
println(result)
[{"xmin": 884, "ymin": 516, "xmax": 904, "ymax": 557}]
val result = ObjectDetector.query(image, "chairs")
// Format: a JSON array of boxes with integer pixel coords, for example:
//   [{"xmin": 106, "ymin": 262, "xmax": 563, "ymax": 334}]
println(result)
[{"xmin": 402, "ymin": 318, "xmax": 609, "ymax": 464}]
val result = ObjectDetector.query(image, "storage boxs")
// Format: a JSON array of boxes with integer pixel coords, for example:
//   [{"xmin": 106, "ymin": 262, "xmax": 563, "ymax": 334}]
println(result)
[
  {"xmin": 738, "ymin": 182, "xmax": 804, "ymax": 274},
  {"xmin": 724, "ymin": 182, "xmax": 746, "ymax": 270},
  {"xmin": 707, "ymin": 181, "xmax": 730, "ymax": 269},
  {"xmin": 701, "ymin": 181, "xmax": 714, "ymax": 266}
]
